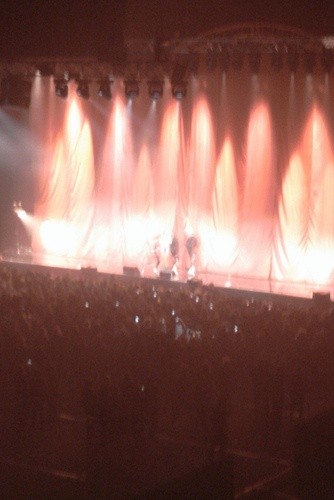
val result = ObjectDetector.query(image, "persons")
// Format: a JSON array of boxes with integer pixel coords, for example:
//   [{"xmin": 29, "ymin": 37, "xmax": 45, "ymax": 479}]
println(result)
[{"xmin": 0, "ymin": 233, "xmax": 334, "ymax": 500}]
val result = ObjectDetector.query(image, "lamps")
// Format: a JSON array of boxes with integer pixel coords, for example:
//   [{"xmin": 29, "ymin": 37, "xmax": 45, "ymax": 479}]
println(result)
[
  {"xmin": 170, "ymin": 80, "xmax": 189, "ymax": 100},
  {"xmin": 121, "ymin": 79, "xmax": 140, "ymax": 98},
  {"xmin": 75, "ymin": 78, "xmax": 92, "ymax": 98},
  {"xmin": 53, "ymin": 79, "xmax": 69, "ymax": 99},
  {"xmin": 97, "ymin": 80, "xmax": 114, "ymax": 101},
  {"xmin": 147, "ymin": 79, "xmax": 164, "ymax": 99}
]
